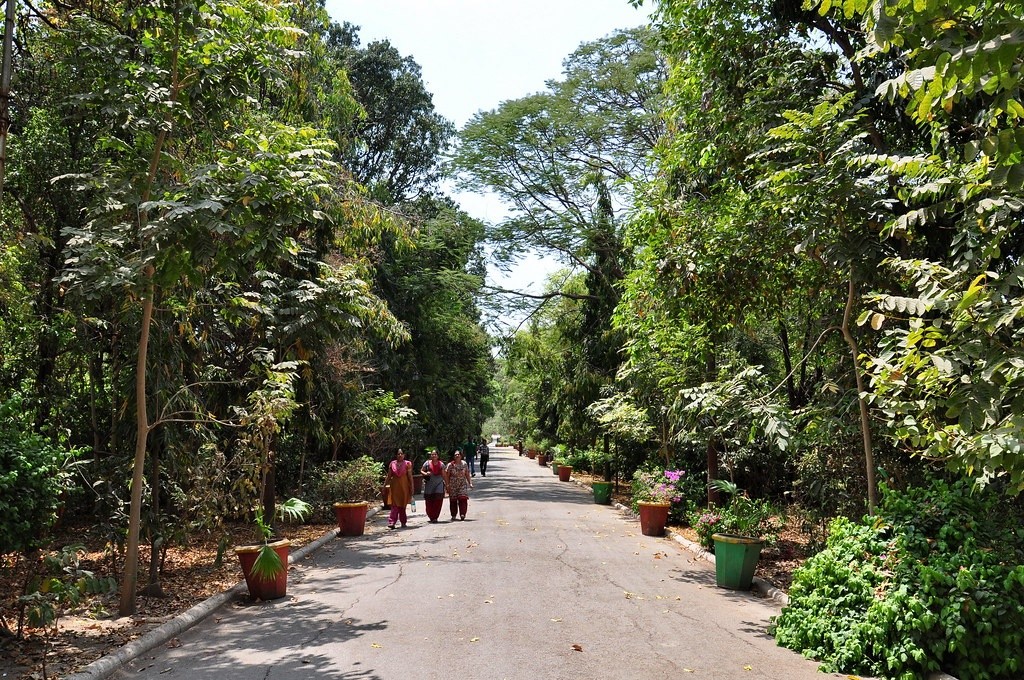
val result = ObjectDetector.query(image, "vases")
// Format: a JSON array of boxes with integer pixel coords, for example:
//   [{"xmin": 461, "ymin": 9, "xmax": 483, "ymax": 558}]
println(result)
[
  {"xmin": 413, "ymin": 475, "xmax": 424, "ymax": 495},
  {"xmin": 637, "ymin": 500, "xmax": 672, "ymax": 535}
]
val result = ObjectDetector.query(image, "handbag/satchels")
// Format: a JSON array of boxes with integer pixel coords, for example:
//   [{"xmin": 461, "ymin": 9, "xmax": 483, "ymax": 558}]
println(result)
[
  {"xmin": 387, "ymin": 488, "xmax": 392, "ymax": 505},
  {"xmin": 424, "ymin": 460, "xmax": 429, "ymax": 481}
]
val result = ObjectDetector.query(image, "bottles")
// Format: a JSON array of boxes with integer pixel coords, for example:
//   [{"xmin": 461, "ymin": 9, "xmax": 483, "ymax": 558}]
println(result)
[{"xmin": 411, "ymin": 495, "xmax": 416, "ymax": 513}]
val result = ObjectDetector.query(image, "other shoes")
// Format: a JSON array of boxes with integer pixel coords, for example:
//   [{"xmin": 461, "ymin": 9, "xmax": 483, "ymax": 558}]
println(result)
[
  {"xmin": 388, "ymin": 524, "xmax": 395, "ymax": 529},
  {"xmin": 402, "ymin": 523, "xmax": 407, "ymax": 528},
  {"xmin": 461, "ymin": 515, "xmax": 465, "ymax": 520},
  {"xmin": 431, "ymin": 518, "xmax": 437, "ymax": 522},
  {"xmin": 452, "ymin": 516, "xmax": 455, "ymax": 520},
  {"xmin": 472, "ymin": 474, "xmax": 475, "ymax": 477},
  {"xmin": 481, "ymin": 471, "xmax": 485, "ymax": 476}
]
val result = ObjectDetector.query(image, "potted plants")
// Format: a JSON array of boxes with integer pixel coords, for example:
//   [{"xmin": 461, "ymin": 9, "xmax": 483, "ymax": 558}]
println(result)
[
  {"xmin": 526, "ymin": 434, "xmax": 784, "ymax": 590},
  {"xmin": 234, "ymin": 496, "xmax": 314, "ymax": 602},
  {"xmin": 373, "ymin": 461, "xmax": 391, "ymax": 509},
  {"xmin": 310, "ymin": 455, "xmax": 387, "ymax": 535}
]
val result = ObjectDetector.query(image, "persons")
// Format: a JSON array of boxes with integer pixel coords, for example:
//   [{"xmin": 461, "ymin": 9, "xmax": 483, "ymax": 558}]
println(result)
[
  {"xmin": 420, "ymin": 448, "xmax": 447, "ymax": 523},
  {"xmin": 478, "ymin": 439, "xmax": 490, "ymax": 477},
  {"xmin": 382, "ymin": 448, "xmax": 414, "ymax": 529},
  {"xmin": 473, "ymin": 437, "xmax": 478, "ymax": 451},
  {"xmin": 445, "ymin": 447, "xmax": 474, "ymax": 521},
  {"xmin": 463, "ymin": 436, "xmax": 478, "ymax": 477}
]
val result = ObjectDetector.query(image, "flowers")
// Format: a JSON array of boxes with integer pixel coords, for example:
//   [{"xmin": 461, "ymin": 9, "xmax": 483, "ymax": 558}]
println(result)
[{"xmin": 641, "ymin": 468, "xmax": 687, "ymax": 502}]
